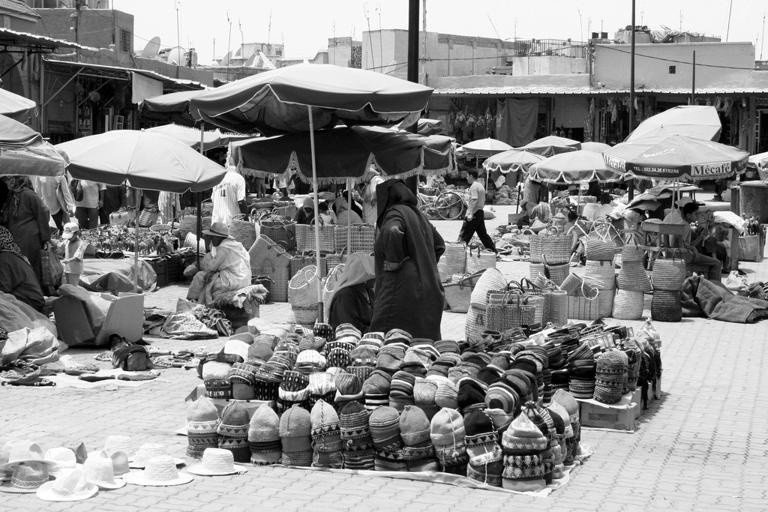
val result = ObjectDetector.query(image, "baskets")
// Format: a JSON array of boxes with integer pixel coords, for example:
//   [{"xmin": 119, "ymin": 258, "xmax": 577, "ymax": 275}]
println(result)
[
  {"xmin": 437, "ymin": 226, "xmax": 653, "ymax": 341},
  {"xmin": 739, "ymin": 235, "xmax": 760, "ymax": 260},
  {"xmin": 231, "ymin": 207, "xmax": 375, "ymax": 324},
  {"xmin": 139, "ymin": 207, "xmax": 160, "ymax": 225},
  {"xmin": 651, "ymin": 247, "xmax": 686, "ymax": 290},
  {"xmin": 652, "ymin": 289, "xmax": 682, "ymax": 321},
  {"xmin": 110, "ymin": 212, "xmax": 131, "ymax": 227}
]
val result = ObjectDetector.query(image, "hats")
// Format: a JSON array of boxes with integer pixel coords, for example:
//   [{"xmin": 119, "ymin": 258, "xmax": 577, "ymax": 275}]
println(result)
[
  {"xmin": 202, "ymin": 222, "xmax": 235, "ymax": 239},
  {"xmin": 569, "ymin": 316, "xmax": 661, "ymax": 405},
  {"xmin": 0, "ymin": 439, "xmax": 192, "ymax": 503},
  {"xmin": 187, "ymin": 447, "xmax": 247, "ymax": 476}
]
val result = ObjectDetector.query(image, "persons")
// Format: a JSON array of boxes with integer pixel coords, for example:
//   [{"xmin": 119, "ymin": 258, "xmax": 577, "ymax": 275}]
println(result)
[
  {"xmin": 39, "ymin": 175, "xmax": 182, "ymax": 285},
  {"xmin": 56, "ymin": 221, "xmax": 85, "ymax": 286},
  {"xmin": 457, "ymin": 171, "xmax": 495, "ymax": 251},
  {"xmin": 496, "ymin": 177, "xmax": 615, "ymax": 227},
  {"xmin": 0, "ymin": 226, "xmax": 44, "ymax": 312},
  {"xmin": 189, "ymin": 222, "xmax": 251, "ymax": 297},
  {"xmin": 1, "ymin": 176, "xmax": 51, "ymax": 297},
  {"xmin": 329, "ymin": 179, "xmax": 446, "ymax": 340},
  {"xmin": 328, "ymin": 253, "xmax": 377, "ymax": 333},
  {"xmin": 651, "ymin": 196, "xmax": 730, "ymax": 279}
]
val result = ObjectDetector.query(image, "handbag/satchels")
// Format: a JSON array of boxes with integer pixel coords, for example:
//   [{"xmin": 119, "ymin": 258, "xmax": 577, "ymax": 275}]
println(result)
[{"xmin": 40, "ymin": 251, "xmax": 63, "ymax": 284}]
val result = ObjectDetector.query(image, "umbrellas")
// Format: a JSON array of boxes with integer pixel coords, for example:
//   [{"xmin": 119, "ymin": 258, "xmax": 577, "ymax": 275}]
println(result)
[{"xmin": 136, "ymin": 62, "xmax": 433, "ymax": 322}]
